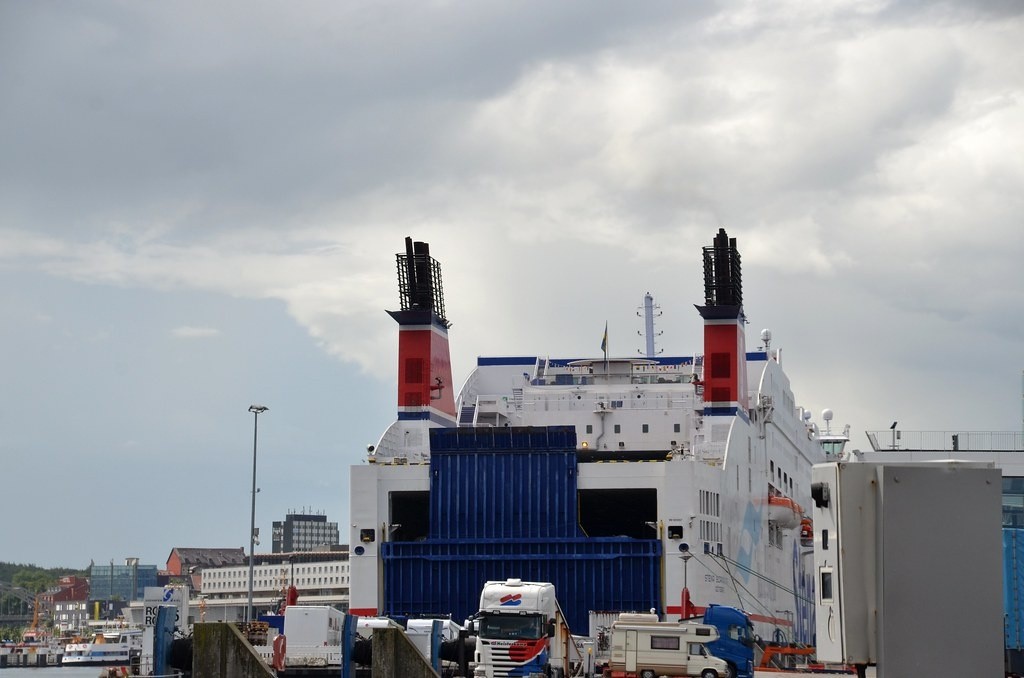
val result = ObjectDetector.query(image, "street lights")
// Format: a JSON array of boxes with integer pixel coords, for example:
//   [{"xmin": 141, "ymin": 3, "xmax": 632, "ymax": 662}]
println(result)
[{"xmin": 246, "ymin": 403, "xmax": 270, "ymax": 626}]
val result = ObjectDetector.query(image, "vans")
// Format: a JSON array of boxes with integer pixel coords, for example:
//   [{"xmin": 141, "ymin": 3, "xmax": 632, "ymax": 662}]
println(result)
[{"xmin": 605, "ymin": 612, "xmax": 730, "ymax": 677}]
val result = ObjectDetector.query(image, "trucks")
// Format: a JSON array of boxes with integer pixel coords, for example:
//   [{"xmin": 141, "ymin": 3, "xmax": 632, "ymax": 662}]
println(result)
[
  {"xmin": 593, "ymin": 601, "xmax": 756, "ymax": 678},
  {"xmin": 466, "ymin": 576, "xmax": 597, "ymax": 678}
]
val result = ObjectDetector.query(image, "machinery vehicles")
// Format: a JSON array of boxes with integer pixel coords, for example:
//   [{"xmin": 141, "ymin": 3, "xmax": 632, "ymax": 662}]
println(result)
[{"xmin": 0, "ymin": 579, "xmax": 55, "ymax": 643}]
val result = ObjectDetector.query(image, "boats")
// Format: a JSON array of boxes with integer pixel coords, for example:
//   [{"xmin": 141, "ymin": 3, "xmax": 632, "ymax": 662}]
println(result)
[{"xmin": 61, "ymin": 628, "xmax": 143, "ymax": 667}]
